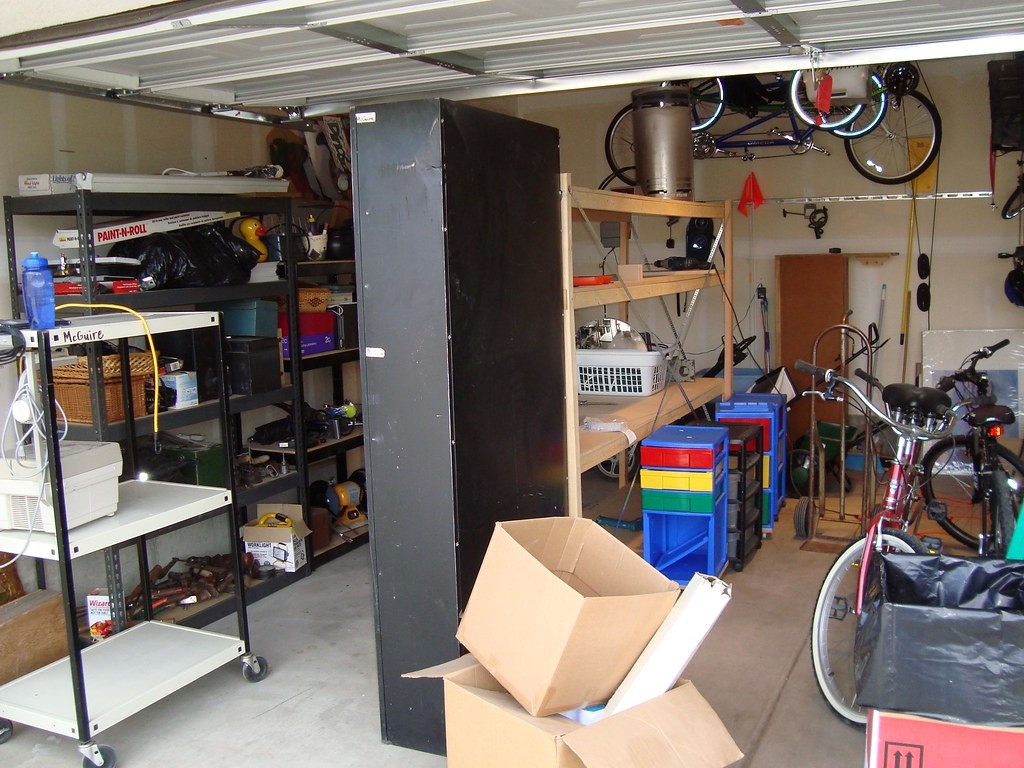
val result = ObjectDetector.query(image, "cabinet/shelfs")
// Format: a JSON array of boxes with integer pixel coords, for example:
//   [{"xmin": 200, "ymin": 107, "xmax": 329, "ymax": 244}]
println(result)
[
  {"xmin": 560, "ymin": 171, "xmax": 732, "ymax": 547},
  {"xmin": 640, "ymin": 393, "xmax": 787, "ymax": 589},
  {"xmin": 0, "ymin": 309, "xmax": 267, "ymax": 768},
  {"xmin": 4, "ymin": 173, "xmax": 370, "ymax": 650}
]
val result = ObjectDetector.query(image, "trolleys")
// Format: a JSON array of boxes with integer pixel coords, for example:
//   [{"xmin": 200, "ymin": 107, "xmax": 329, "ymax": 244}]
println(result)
[{"xmin": 0, "ymin": 309, "xmax": 273, "ymax": 768}]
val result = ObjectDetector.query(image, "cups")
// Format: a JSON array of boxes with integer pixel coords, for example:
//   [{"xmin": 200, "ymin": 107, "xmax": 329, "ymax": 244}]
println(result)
[{"xmin": 302, "ymin": 233, "xmax": 328, "ymax": 261}]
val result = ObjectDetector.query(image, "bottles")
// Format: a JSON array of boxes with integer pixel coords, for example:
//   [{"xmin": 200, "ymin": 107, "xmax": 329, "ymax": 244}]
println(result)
[{"xmin": 21, "ymin": 252, "xmax": 56, "ymax": 329}]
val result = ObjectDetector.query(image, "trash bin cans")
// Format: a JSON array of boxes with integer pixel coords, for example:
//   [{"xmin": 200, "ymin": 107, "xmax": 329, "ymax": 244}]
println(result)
[{"xmin": 788, "ymin": 420, "xmax": 859, "ymax": 485}]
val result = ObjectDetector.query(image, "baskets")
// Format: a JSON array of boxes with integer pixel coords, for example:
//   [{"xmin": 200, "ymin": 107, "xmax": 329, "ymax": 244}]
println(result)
[
  {"xmin": 37, "ymin": 375, "xmax": 148, "ymax": 429},
  {"xmin": 51, "ymin": 350, "xmax": 159, "ymax": 380},
  {"xmin": 575, "ymin": 344, "xmax": 676, "ymax": 397},
  {"xmin": 261, "ymin": 287, "xmax": 330, "ymax": 312}
]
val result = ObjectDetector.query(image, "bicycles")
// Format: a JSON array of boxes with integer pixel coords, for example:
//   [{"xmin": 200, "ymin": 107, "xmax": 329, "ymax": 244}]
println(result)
[
  {"xmin": 603, "ymin": 60, "xmax": 944, "ymax": 192},
  {"xmin": 995, "ymin": 173, "xmax": 1024, "ymax": 302},
  {"xmin": 930, "ymin": 338, "xmax": 1024, "ymax": 567},
  {"xmin": 791, "ymin": 355, "xmax": 958, "ymax": 733}
]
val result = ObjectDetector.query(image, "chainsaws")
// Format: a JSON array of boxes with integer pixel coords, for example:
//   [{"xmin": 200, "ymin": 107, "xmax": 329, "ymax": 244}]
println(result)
[{"xmin": 307, "ymin": 478, "xmax": 368, "ymax": 533}]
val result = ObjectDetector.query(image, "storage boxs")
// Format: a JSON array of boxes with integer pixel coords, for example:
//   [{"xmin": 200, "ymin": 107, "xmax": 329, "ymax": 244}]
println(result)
[
  {"xmin": 311, "ymin": 508, "xmax": 330, "ymax": 549},
  {"xmin": 154, "ymin": 371, "xmax": 198, "ymax": 410},
  {"xmin": 277, "ymin": 310, "xmax": 336, "ymax": 359},
  {"xmin": 52, "ymin": 212, "xmax": 240, "ymax": 248},
  {"xmin": 87, "ymin": 587, "xmax": 125, "ymax": 643},
  {"xmin": 155, "ymin": 299, "xmax": 281, "ymax": 403},
  {"xmin": 574, "ymin": 343, "xmax": 676, "ymax": 397},
  {"xmin": 239, "ymin": 504, "xmax": 313, "ymax": 573},
  {"xmin": 329, "ymin": 292, "xmax": 353, "ymax": 305},
  {"xmin": 400, "ymin": 517, "xmax": 744, "ymax": 768},
  {"xmin": 618, "ymin": 265, "xmax": 644, "ymax": 281},
  {"xmin": 19, "ymin": 174, "xmax": 76, "ymax": 197},
  {"xmin": 852, "ymin": 554, "xmax": 1024, "ymax": 768}
]
